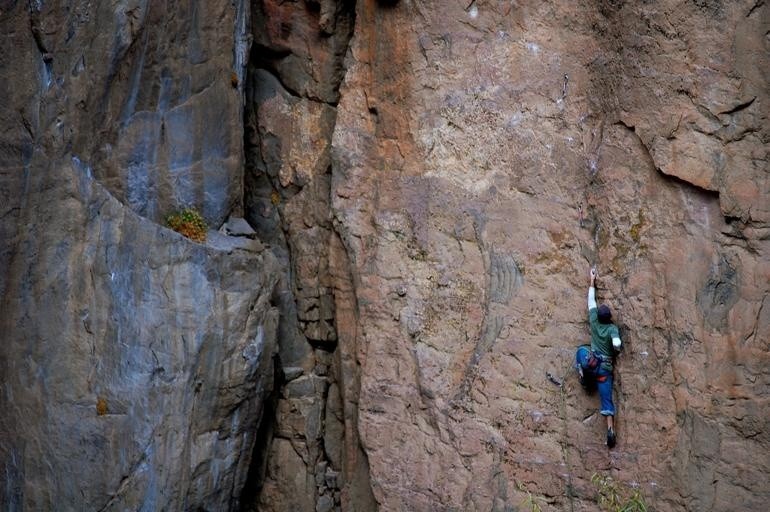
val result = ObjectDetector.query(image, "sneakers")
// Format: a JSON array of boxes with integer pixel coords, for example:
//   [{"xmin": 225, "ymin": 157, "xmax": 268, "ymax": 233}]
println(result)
[{"xmin": 607, "ymin": 426, "xmax": 616, "ymax": 448}]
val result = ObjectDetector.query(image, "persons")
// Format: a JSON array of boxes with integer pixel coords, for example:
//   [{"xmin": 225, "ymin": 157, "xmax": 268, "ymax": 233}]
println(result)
[{"xmin": 576, "ymin": 269, "xmax": 624, "ymax": 448}]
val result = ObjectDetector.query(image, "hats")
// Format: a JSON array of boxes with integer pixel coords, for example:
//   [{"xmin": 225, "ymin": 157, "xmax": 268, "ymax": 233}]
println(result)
[{"xmin": 598, "ymin": 305, "xmax": 612, "ymax": 320}]
